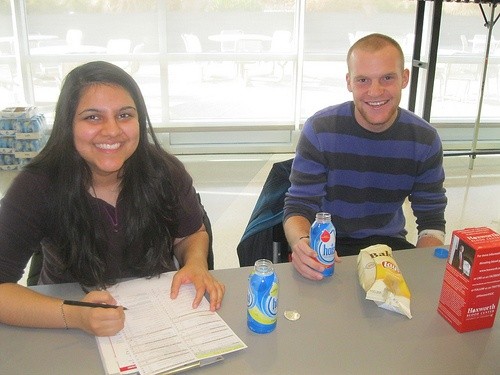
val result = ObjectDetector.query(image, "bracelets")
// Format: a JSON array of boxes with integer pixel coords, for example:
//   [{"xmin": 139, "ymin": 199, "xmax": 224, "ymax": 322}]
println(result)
[
  {"xmin": 60, "ymin": 300, "xmax": 69, "ymax": 330},
  {"xmin": 299, "ymin": 236, "xmax": 309, "ymax": 240}
]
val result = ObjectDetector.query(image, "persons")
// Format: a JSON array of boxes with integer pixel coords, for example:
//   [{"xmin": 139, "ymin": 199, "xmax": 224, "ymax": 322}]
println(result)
[
  {"xmin": 452, "ymin": 244, "xmax": 465, "ymax": 273},
  {"xmin": 0, "ymin": 61, "xmax": 224, "ymax": 336},
  {"xmin": 284, "ymin": 34, "xmax": 448, "ymax": 280}
]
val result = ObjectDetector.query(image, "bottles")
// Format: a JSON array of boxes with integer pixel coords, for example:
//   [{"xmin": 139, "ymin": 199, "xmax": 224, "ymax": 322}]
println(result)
[
  {"xmin": 0, "ymin": 106, "xmax": 50, "ymax": 171},
  {"xmin": 247, "ymin": 259, "xmax": 279, "ymax": 334},
  {"xmin": 310, "ymin": 212, "xmax": 337, "ymax": 280}
]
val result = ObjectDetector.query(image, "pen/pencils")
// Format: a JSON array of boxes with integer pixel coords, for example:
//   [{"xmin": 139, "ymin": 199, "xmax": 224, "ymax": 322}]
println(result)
[{"xmin": 62, "ymin": 299, "xmax": 129, "ymax": 311}]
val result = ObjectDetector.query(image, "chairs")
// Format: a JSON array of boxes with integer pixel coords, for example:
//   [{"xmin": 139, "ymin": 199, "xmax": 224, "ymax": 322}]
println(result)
[{"xmin": 238, "ymin": 158, "xmax": 292, "ymax": 267}]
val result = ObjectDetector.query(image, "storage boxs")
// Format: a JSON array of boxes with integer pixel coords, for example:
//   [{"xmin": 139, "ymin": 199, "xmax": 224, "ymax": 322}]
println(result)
[{"xmin": 438, "ymin": 227, "xmax": 500, "ymax": 333}]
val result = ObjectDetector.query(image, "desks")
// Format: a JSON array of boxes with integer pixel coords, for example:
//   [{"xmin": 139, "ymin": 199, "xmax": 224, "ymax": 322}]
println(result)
[{"xmin": 0, "ymin": 246, "xmax": 500, "ymax": 375}]
